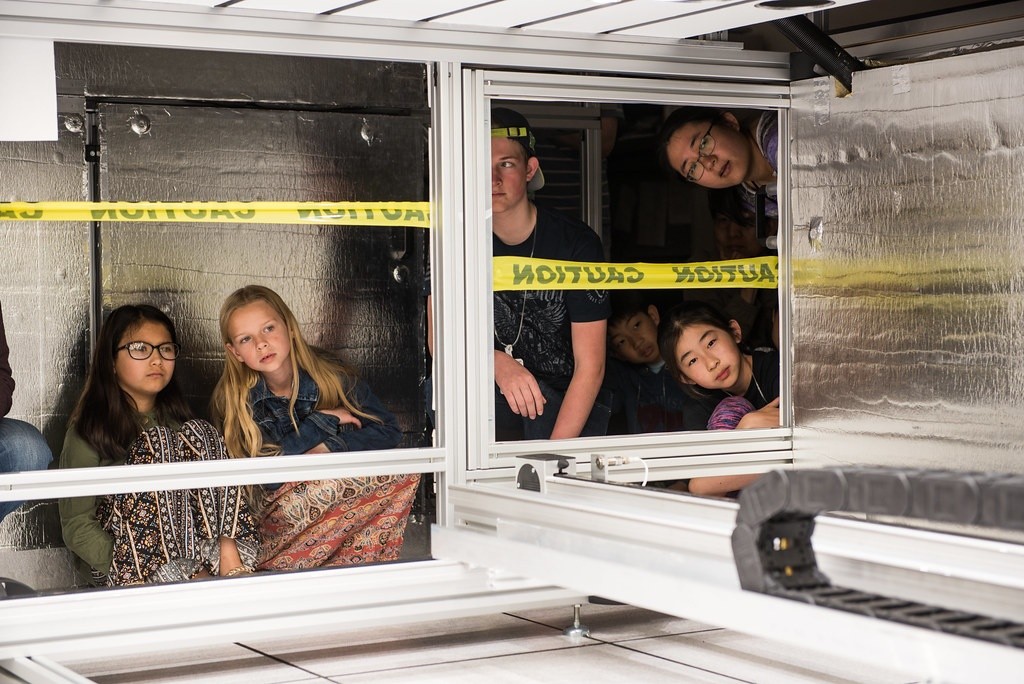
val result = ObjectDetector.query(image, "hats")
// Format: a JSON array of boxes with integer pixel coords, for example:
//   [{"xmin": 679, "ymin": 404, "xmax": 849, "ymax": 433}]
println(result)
[{"xmin": 491, "ymin": 107, "xmax": 545, "ymax": 192}]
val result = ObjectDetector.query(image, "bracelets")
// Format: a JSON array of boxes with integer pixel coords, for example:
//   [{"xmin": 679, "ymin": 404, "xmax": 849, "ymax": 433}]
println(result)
[{"xmin": 225, "ymin": 566, "xmax": 254, "ymax": 577}]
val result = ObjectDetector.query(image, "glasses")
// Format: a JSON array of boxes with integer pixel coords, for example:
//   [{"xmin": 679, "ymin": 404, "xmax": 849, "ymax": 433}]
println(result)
[
  {"xmin": 118, "ymin": 340, "xmax": 181, "ymax": 360},
  {"xmin": 685, "ymin": 113, "xmax": 723, "ymax": 180}
]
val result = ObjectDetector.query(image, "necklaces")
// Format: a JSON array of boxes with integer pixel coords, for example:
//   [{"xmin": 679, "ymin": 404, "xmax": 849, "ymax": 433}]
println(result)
[
  {"xmin": 634, "ymin": 363, "xmax": 667, "ymax": 433},
  {"xmin": 723, "ymin": 354, "xmax": 768, "ymax": 409}
]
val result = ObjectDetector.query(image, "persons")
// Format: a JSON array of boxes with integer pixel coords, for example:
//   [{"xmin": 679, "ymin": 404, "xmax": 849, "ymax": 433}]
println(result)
[
  {"xmin": 658, "ymin": 106, "xmax": 778, "ymax": 259},
  {"xmin": 681, "ymin": 185, "xmax": 778, "ymax": 352},
  {"xmin": 0, "ymin": 300, "xmax": 53, "ymax": 526},
  {"xmin": 423, "ymin": 108, "xmax": 614, "ymax": 440},
  {"xmin": 59, "ymin": 304, "xmax": 262, "ymax": 588},
  {"xmin": 606, "ymin": 288, "xmax": 710, "ymax": 491},
  {"xmin": 214, "ymin": 285, "xmax": 425, "ymax": 571},
  {"xmin": 656, "ymin": 300, "xmax": 778, "ymax": 495}
]
